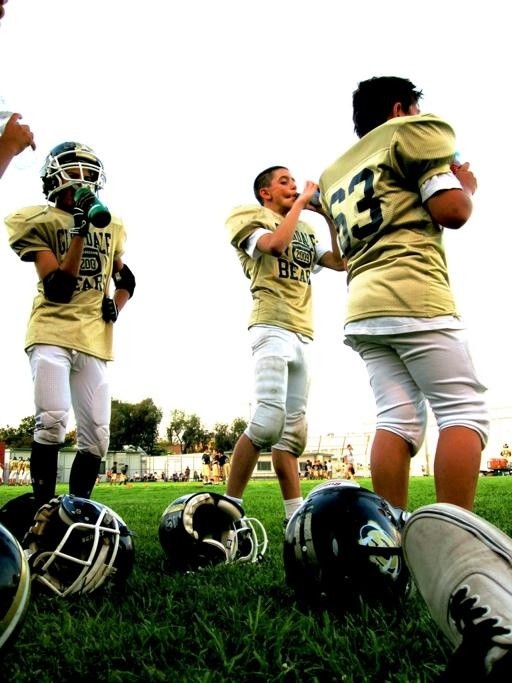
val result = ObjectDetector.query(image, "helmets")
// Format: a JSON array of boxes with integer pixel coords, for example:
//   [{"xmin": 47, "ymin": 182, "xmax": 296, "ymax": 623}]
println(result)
[
  {"xmin": 158, "ymin": 492, "xmax": 267, "ymax": 574},
  {"xmin": 285, "ymin": 478, "xmax": 412, "ymax": 623},
  {"xmin": 21, "ymin": 495, "xmax": 134, "ymax": 601},
  {"xmin": 0, "ymin": 522, "xmax": 31, "ymax": 653},
  {"xmin": 39, "ymin": 142, "xmax": 106, "ymax": 201}
]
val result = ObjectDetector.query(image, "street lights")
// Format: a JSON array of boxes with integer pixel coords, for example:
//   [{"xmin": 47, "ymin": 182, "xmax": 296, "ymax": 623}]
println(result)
[{"xmin": 317, "ymin": 431, "xmax": 337, "ymax": 452}]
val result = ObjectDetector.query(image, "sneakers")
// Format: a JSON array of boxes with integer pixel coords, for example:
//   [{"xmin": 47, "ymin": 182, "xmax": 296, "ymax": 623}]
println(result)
[{"xmin": 401, "ymin": 503, "xmax": 512, "ymax": 674}]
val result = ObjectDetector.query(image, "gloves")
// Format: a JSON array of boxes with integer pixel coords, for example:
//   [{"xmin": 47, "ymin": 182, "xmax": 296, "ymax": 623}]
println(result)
[
  {"xmin": 70, "ymin": 193, "xmax": 95, "ymax": 237},
  {"xmin": 101, "ymin": 298, "xmax": 118, "ymax": 323}
]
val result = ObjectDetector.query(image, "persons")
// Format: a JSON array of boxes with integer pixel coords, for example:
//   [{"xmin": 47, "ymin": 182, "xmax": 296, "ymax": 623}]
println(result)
[
  {"xmin": 0, "ymin": 112, "xmax": 36, "ymax": 182},
  {"xmin": 318, "ymin": 75, "xmax": 490, "ymax": 514},
  {"xmin": 4, "ymin": 142, "xmax": 135, "ymax": 501},
  {"xmin": 501, "ymin": 444, "xmax": 512, "ymax": 476},
  {"xmin": 221, "ymin": 166, "xmax": 348, "ymax": 528}
]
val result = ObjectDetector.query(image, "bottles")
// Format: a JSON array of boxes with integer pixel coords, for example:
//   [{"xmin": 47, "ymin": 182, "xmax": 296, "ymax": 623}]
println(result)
[
  {"xmin": 74, "ymin": 184, "xmax": 111, "ymax": 228},
  {"xmin": 1, "ymin": 99, "xmax": 46, "ymax": 170},
  {"xmin": 450, "ymin": 153, "xmax": 464, "ymax": 175},
  {"xmin": 294, "ymin": 187, "xmax": 323, "ymax": 209}
]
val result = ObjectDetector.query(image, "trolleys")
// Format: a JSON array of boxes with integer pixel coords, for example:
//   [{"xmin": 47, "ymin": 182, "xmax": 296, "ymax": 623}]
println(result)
[{"xmin": 487, "ymin": 456, "xmax": 512, "ymax": 476}]
